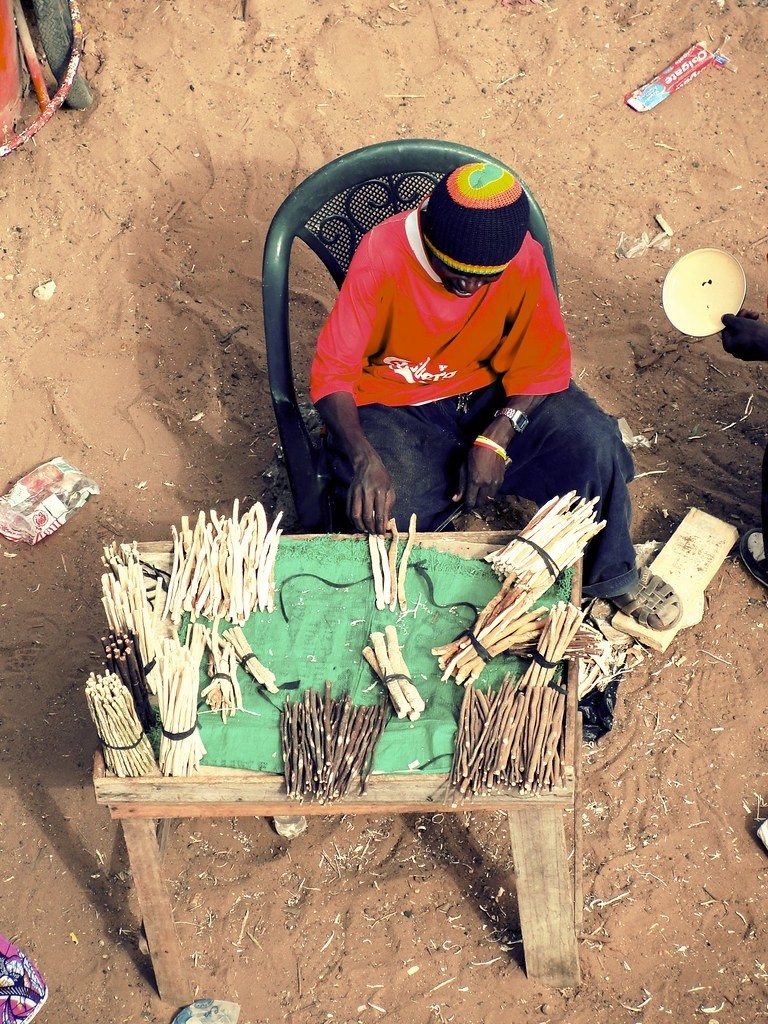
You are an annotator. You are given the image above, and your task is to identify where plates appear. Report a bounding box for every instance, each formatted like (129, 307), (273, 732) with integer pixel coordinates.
(662, 249), (746, 337)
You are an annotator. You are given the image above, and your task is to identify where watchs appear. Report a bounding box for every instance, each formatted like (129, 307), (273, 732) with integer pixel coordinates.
(494, 408), (530, 434)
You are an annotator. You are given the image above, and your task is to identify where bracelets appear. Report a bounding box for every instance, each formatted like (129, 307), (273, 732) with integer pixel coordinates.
(474, 435), (512, 465)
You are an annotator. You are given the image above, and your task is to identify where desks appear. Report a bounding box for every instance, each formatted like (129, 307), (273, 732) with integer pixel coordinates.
(92, 530), (585, 986)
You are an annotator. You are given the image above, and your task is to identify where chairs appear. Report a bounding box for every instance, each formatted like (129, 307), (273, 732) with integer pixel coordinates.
(260, 137), (560, 529)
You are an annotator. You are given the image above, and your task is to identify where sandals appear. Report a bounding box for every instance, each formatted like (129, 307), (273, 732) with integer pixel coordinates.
(611, 566), (683, 631)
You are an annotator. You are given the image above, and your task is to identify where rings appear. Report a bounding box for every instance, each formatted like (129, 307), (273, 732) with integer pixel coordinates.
(486, 495), (494, 500)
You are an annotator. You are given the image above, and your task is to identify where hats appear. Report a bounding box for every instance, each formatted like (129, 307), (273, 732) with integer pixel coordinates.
(423, 161), (530, 278)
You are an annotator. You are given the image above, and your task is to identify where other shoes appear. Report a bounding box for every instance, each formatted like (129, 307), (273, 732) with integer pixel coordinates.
(739, 527), (768, 588)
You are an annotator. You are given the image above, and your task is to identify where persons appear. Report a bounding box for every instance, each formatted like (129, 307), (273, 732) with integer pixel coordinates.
(721, 308), (768, 587)
(307, 161), (685, 635)
